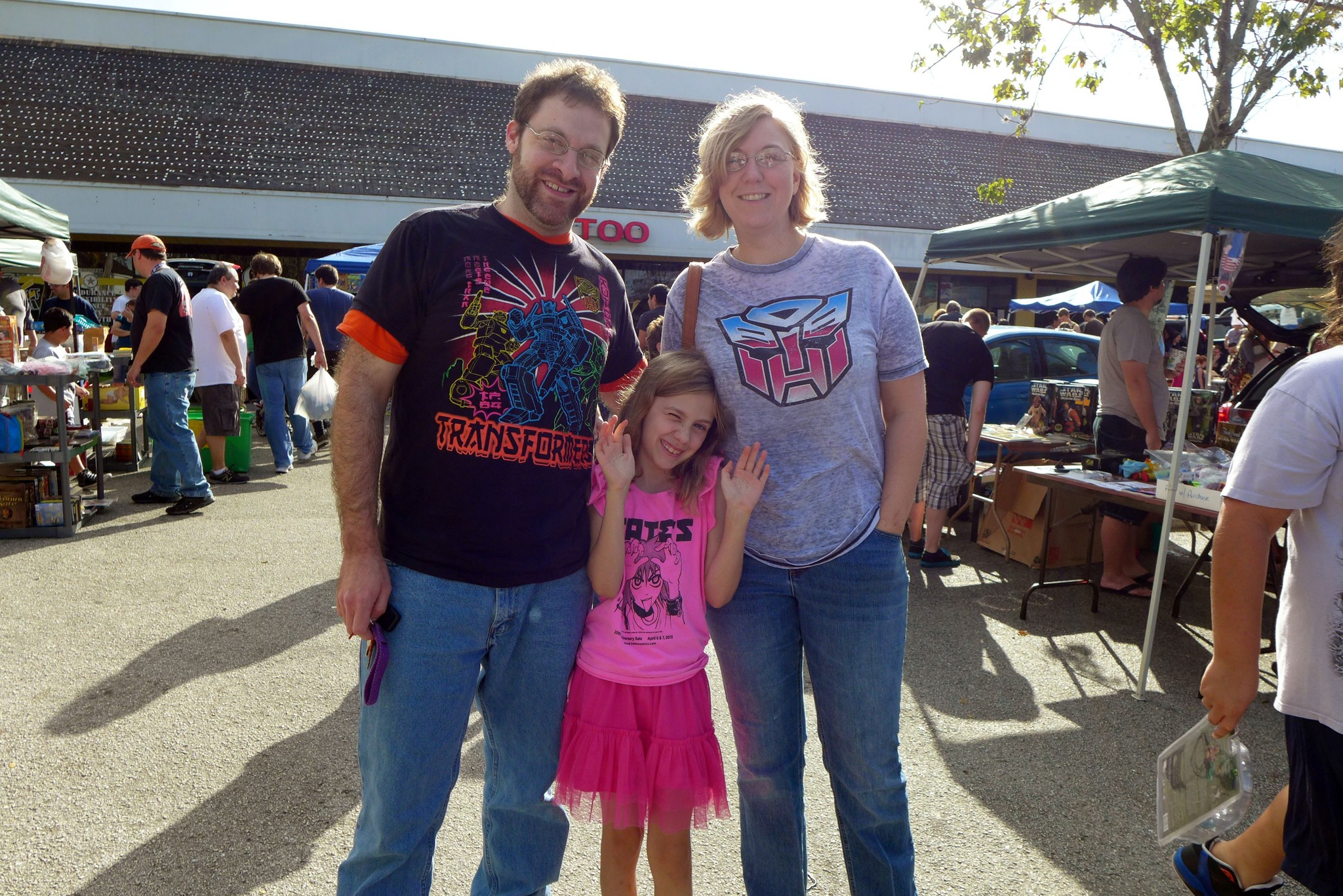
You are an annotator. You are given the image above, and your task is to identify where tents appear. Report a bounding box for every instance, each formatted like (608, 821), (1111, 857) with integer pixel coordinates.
(303, 242), (386, 292)
(910, 149), (1343, 701)
(1006, 280), (1126, 322)
(0, 238), (82, 298)
(0, 178), (83, 427)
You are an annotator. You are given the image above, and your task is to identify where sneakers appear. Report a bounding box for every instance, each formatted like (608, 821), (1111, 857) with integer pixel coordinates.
(132, 490), (181, 503)
(209, 467), (250, 484)
(275, 464), (293, 473)
(165, 495), (216, 514)
(1172, 836), (1283, 896)
(297, 440), (318, 460)
(316, 434), (329, 450)
(77, 468), (112, 490)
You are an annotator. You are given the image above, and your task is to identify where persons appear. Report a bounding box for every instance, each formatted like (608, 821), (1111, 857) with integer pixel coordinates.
(661, 87), (931, 895)
(1094, 255), (1170, 599)
(645, 314), (664, 361)
(1188, 395), (1209, 434)
(0, 253), (356, 489)
(330, 61), (651, 896)
(636, 284), (671, 353)
(554, 346), (770, 896)
(930, 299), (1323, 404)
(121, 235), (215, 516)
(905, 308), (995, 569)
(1172, 221), (1343, 896)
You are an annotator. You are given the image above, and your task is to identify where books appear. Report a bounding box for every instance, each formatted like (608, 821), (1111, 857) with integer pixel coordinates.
(0, 465), (114, 529)
(1028, 378), (1100, 436)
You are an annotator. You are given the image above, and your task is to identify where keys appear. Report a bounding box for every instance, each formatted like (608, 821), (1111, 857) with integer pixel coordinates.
(349, 603), (403, 669)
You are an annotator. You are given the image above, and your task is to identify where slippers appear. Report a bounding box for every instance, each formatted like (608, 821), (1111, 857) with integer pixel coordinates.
(1133, 572), (1167, 587)
(1099, 581), (1152, 599)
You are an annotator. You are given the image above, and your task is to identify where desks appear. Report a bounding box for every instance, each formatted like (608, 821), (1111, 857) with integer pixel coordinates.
(1012, 464), (1287, 623)
(945, 423), (1096, 563)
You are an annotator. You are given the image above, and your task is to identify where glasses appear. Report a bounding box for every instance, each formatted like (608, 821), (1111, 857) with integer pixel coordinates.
(725, 147), (798, 171)
(524, 122), (610, 170)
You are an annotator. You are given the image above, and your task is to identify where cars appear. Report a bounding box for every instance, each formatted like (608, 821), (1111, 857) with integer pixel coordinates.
(963, 323), (1100, 461)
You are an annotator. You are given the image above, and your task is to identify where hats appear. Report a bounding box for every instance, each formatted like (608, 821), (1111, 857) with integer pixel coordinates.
(126, 235), (167, 259)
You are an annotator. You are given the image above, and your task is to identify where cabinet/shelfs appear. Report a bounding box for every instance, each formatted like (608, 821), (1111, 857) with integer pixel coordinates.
(78, 370), (150, 472)
(0, 369), (107, 538)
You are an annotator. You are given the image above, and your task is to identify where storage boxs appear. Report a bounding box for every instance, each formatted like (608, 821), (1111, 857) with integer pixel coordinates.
(0, 314), (21, 363)
(187, 406), (244, 413)
(1082, 452), (1137, 475)
(152, 412), (255, 473)
(83, 325), (110, 353)
(1160, 386), (1221, 444)
(90, 426), (128, 446)
(1156, 477), (1223, 512)
(976, 458), (1163, 571)
